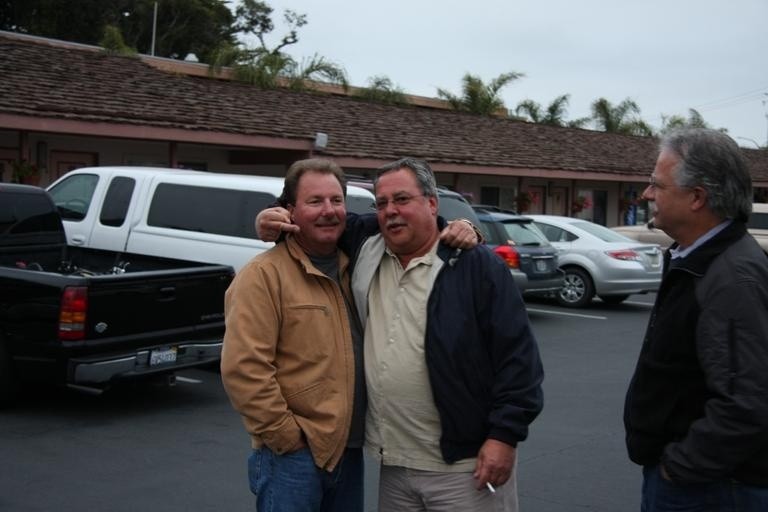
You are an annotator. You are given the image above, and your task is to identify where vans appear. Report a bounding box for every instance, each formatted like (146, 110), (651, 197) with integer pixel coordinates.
(44, 165), (379, 276)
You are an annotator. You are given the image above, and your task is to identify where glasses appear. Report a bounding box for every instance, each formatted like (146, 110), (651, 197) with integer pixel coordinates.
(368, 192), (427, 211)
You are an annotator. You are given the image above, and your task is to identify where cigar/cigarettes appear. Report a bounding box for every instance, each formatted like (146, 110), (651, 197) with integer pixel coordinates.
(486, 479), (499, 496)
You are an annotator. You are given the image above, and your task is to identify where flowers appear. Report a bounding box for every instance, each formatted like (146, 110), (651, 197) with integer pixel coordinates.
(577, 192), (592, 208)
(511, 190), (540, 206)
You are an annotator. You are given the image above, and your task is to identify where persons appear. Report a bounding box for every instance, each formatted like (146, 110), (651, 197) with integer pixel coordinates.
(622, 126), (768, 512)
(214, 158), (485, 512)
(252, 160), (545, 511)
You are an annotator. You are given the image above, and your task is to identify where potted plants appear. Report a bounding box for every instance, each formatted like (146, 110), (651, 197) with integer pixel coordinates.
(8, 158), (49, 187)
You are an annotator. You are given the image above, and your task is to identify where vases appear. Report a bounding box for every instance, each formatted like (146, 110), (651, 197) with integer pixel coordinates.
(518, 202), (528, 211)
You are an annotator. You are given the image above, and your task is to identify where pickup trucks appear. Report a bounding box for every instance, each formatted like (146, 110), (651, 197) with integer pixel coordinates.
(0, 183), (236, 395)
